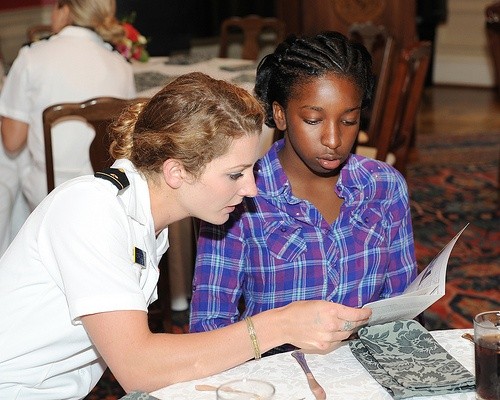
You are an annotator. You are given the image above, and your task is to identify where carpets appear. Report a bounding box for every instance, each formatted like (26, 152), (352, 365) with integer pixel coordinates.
(407, 129), (500, 329)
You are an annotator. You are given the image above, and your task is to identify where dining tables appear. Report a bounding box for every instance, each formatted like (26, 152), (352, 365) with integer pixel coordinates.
(150, 322), (500, 400)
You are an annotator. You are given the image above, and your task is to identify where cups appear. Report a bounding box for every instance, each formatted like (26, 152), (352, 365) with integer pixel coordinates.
(216, 379), (275, 400)
(473, 310), (500, 400)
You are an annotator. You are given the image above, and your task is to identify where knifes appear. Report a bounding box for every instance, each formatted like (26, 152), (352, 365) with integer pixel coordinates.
(292, 350), (327, 400)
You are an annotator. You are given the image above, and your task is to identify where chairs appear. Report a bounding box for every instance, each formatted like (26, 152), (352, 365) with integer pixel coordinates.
(362, 37), (434, 169)
(220, 14), (290, 66)
(345, 22), (399, 130)
(40, 93), (146, 197)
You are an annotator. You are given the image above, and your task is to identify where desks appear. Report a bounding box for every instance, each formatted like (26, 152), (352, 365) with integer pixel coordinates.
(132, 53), (260, 96)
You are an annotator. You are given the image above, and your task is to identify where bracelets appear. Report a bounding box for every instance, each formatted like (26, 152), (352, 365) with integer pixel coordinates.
(246, 316), (262, 360)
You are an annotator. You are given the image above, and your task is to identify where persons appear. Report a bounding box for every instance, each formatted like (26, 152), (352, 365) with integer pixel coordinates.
(190, 34), (417, 350)
(0, 0), (136, 214)
(0, 71), (373, 399)
(382, 0), (447, 89)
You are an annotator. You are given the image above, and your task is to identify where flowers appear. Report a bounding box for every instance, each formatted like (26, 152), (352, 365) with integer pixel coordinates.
(111, 18), (150, 62)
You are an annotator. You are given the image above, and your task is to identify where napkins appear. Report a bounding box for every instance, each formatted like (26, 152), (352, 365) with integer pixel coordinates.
(134, 71), (178, 91)
(165, 54), (211, 64)
(349, 317), (476, 400)
(218, 63), (257, 70)
(233, 72), (256, 82)
(119, 388), (161, 400)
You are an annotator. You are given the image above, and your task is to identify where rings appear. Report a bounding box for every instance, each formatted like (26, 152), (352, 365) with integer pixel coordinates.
(344, 320), (351, 331)
